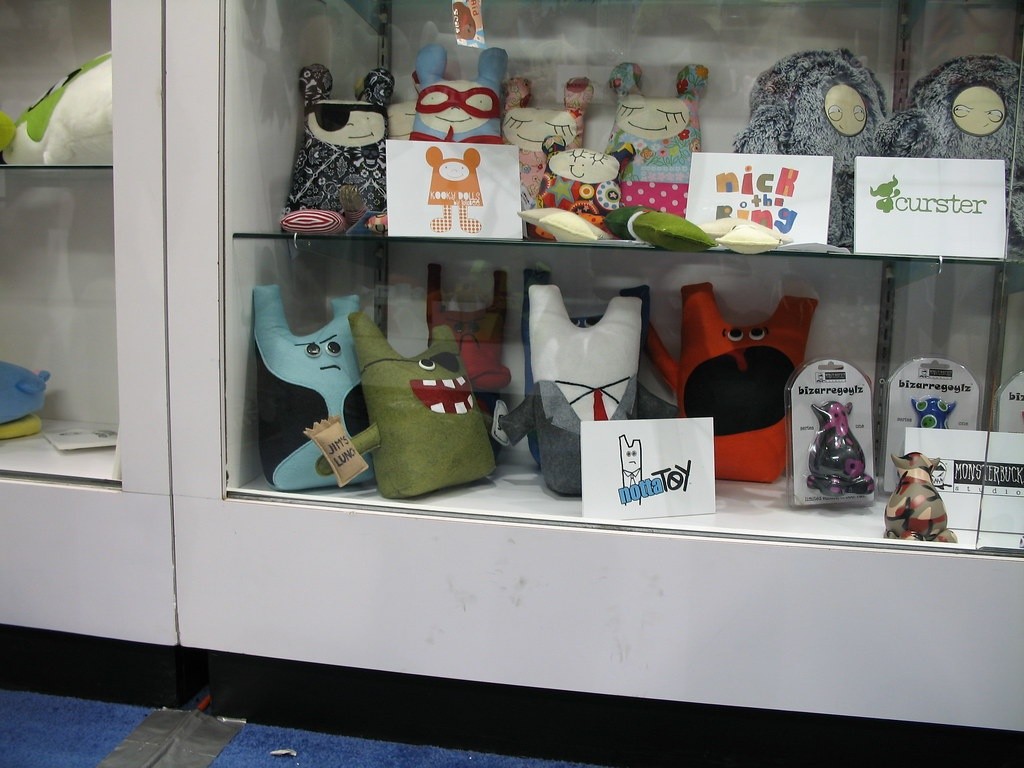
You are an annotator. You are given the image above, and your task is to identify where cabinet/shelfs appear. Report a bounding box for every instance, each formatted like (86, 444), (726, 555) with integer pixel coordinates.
(2, 0), (1024, 729)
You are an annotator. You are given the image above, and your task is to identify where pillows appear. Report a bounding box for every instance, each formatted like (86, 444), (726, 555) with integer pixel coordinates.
(3, 47), (114, 166)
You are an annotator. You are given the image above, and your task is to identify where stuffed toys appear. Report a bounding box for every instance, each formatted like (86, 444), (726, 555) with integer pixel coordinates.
(278, 45), (1024, 253)
(0, 54), (113, 166)
(255, 262), (818, 498)
